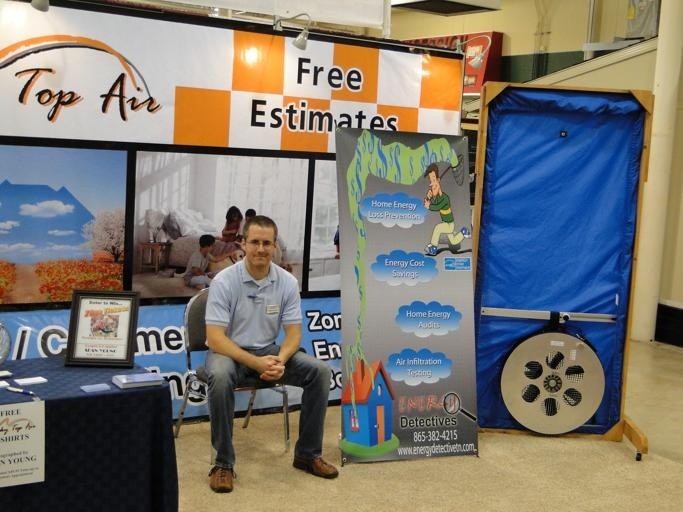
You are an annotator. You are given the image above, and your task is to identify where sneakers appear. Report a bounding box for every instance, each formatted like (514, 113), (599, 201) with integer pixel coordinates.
(209, 464), (236, 493)
(424, 243), (438, 256)
(460, 226), (472, 239)
(293, 454), (339, 479)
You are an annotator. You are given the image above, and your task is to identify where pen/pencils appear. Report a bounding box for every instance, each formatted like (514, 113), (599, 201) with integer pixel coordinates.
(6, 386), (36, 396)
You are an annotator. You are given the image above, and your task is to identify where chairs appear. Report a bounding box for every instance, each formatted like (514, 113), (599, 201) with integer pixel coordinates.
(171, 287), (289, 461)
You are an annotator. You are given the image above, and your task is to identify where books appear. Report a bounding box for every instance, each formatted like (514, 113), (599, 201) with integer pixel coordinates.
(110, 373), (165, 388)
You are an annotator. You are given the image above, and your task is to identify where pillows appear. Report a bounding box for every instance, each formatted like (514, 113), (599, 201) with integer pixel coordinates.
(163, 208), (217, 240)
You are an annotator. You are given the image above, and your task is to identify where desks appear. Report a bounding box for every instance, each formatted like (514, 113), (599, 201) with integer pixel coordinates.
(137, 242), (168, 276)
(0, 357), (172, 511)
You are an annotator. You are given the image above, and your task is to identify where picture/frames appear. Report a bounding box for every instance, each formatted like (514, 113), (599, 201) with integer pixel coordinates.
(0, 135), (341, 311)
(62, 289), (140, 370)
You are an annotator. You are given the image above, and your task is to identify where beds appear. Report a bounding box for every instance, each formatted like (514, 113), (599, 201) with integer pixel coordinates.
(142, 229), (281, 274)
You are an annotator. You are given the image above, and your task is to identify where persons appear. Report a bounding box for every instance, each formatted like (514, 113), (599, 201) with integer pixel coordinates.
(333, 224), (340, 259)
(181, 204), (293, 290)
(204, 215), (339, 490)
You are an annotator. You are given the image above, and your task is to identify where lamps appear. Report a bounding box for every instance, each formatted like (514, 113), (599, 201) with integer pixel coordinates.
(454, 34), (493, 70)
(269, 13), (312, 51)
